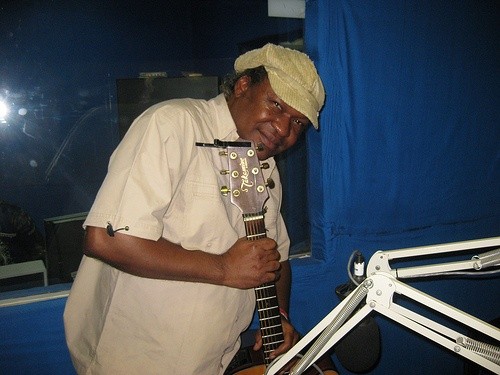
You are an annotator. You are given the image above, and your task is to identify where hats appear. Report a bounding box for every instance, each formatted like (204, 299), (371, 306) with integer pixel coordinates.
(234, 42), (326, 130)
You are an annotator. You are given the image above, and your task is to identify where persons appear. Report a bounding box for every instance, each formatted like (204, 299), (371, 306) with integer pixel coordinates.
(62, 44), (325, 375)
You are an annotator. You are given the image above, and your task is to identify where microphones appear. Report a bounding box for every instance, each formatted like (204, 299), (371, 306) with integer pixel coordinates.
(335, 252), (382, 373)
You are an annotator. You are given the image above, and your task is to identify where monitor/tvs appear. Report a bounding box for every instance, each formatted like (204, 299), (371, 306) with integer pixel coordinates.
(44, 211), (90, 286)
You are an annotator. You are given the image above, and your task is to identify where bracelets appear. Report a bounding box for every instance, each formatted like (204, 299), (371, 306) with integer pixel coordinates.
(279, 307), (289, 321)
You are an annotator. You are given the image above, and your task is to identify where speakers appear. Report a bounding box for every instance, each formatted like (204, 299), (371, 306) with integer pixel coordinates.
(108, 75), (218, 149)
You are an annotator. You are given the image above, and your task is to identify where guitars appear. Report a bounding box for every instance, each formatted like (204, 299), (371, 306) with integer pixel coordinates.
(218, 138), (336, 375)
